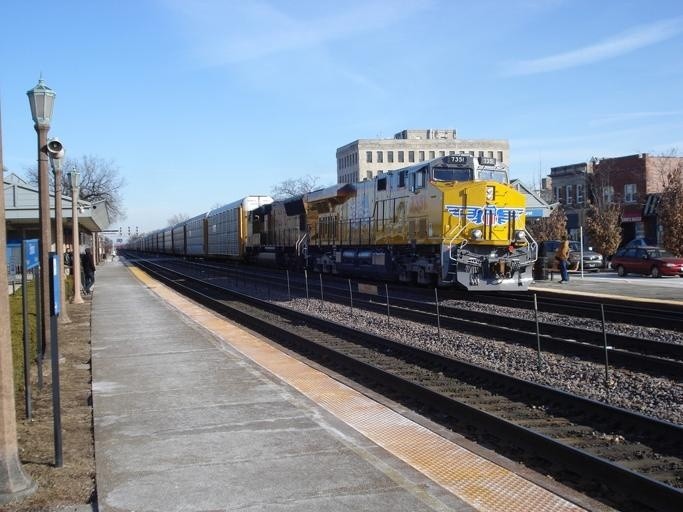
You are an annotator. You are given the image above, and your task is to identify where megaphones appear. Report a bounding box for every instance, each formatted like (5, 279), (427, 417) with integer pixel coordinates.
(47, 137), (63, 152)
(50, 150), (63, 158)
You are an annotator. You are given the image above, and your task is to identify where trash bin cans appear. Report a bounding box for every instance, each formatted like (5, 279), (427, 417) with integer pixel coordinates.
(532, 256), (549, 280)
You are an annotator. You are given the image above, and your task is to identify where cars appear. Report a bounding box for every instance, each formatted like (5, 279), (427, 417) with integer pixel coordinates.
(611, 246), (683, 278)
(538, 240), (603, 269)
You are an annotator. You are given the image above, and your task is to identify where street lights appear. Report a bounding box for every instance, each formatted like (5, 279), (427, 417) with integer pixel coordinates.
(26, 71), (84, 361)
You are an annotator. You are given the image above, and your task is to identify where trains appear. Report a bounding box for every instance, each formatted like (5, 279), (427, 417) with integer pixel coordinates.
(130, 154), (538, 291)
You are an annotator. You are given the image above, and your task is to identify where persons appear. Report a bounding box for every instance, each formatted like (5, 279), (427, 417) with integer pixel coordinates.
(63, 246), (72, 275)
(81, 247), (95, 295)
(554, 236), (569, 282)
